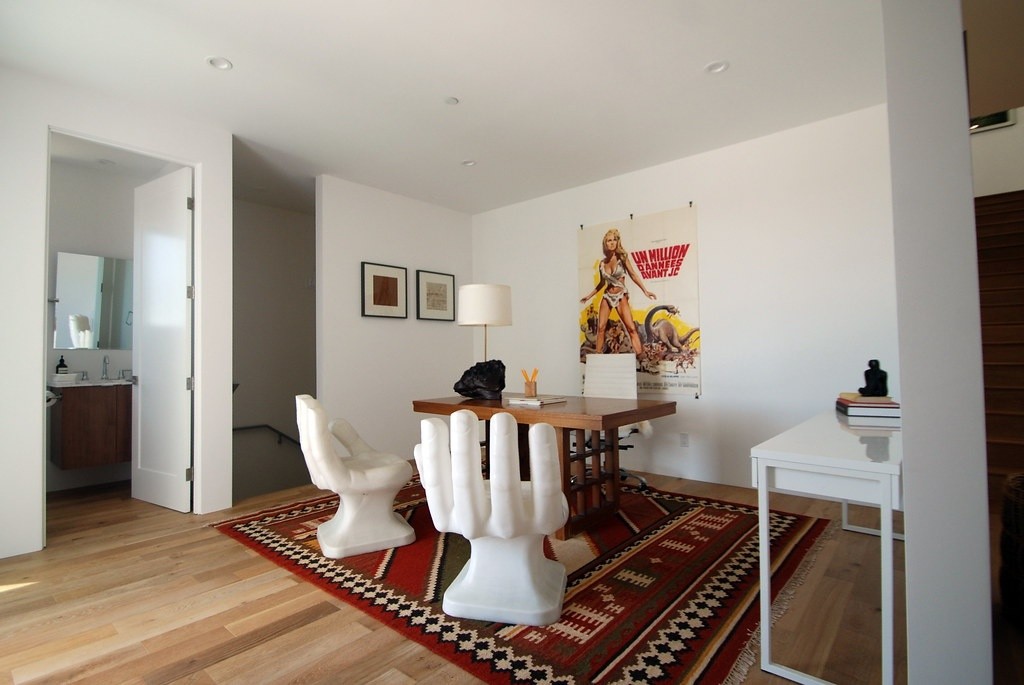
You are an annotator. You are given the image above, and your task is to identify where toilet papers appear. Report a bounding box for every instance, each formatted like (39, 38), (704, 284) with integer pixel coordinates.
(46, 390), (57, 408)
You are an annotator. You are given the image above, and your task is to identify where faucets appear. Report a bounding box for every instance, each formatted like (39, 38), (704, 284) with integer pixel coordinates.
(102, 354), (110, 379)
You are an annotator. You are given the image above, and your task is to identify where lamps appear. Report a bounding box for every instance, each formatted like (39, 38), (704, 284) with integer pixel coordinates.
(458, 284), (513, 362)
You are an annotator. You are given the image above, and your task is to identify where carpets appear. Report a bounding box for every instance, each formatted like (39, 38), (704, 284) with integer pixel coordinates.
(205, 457), (842, 685)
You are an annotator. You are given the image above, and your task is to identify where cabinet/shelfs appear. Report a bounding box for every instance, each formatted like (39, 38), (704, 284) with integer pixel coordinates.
(49, 385), (132, 472)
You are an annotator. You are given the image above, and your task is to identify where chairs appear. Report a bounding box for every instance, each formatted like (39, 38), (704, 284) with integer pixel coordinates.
(414, 409), (570, 626)
(571, 353), (648, 491)
(69, 315), (90, 347)
(295, 394), (417, 559)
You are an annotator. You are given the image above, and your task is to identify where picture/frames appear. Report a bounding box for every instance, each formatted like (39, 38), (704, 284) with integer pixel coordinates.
(361, 261), (407, 319)
(416, 270), (455, 321)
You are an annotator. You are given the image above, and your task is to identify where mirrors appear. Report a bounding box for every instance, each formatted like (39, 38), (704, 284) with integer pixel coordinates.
(53, 251), (134, 350)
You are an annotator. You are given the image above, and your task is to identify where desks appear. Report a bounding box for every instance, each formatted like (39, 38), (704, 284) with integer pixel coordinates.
(751, 409), (903, 685)
(413, 392), (677, 542)
